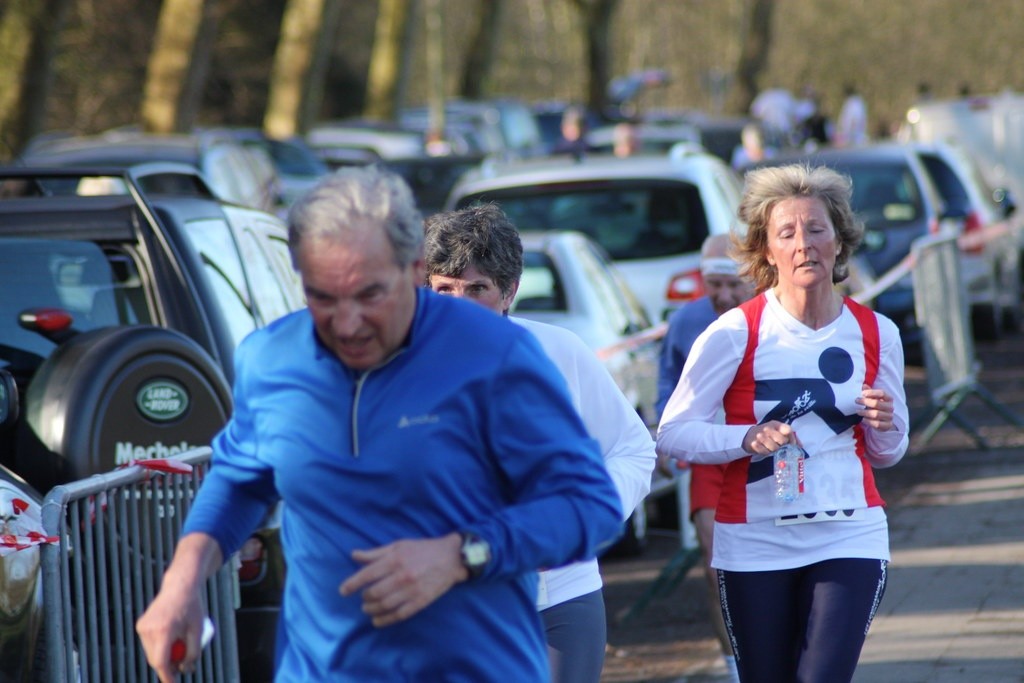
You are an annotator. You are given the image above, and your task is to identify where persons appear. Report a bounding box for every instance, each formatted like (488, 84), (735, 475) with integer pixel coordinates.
(541, 70), (1023, 174)
(134, 161), (624, 683)
(655, 161), (910, 683)
(648, 235), (760, 683)
(419, 200), (657, 682)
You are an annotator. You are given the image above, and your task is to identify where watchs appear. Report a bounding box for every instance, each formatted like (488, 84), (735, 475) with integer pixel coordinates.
(453, 531), (492, 586)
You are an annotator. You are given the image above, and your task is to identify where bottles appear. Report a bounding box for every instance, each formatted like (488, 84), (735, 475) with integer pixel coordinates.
(773, 439), (805, 501)
(170, 616), (215, 663)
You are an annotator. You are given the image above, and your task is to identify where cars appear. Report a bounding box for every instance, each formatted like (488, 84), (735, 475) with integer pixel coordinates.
(0, 97), (1023, 682)
